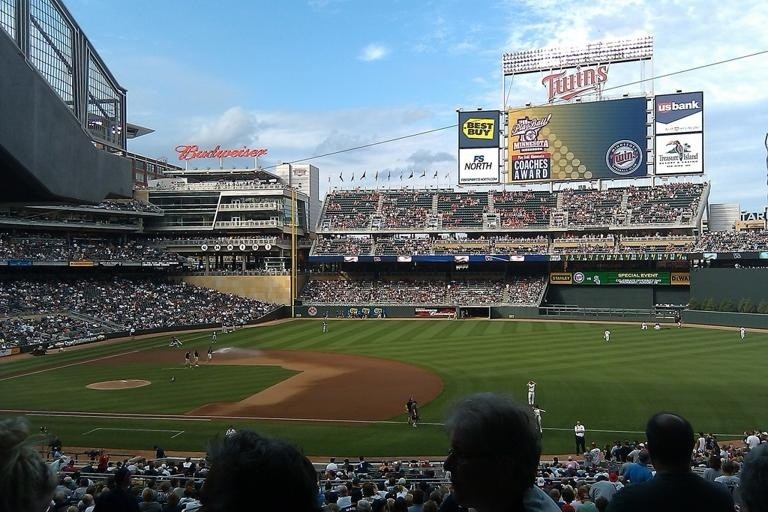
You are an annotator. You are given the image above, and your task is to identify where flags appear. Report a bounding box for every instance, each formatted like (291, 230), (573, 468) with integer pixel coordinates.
(339, 170), (451, 180)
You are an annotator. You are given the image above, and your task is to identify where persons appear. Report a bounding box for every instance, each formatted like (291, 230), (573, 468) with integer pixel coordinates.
(191, 349), (200, 368)
(652, 322), (660, 329)
(603, 328), (611, 344)
(526, 380), (537, 413)
(322, 320), (329, 334)
(738, 325), (745, 342)
(406, 396), (414, 425)
(533, 405), (546, 440)
(206, 346), (214, 363)
(640, 321), (648, 330)
(675, 321), (681, 328)
(184, 351), (190, 368)
(441, 387), (559, 512)
(411, 401), (418, 428)
